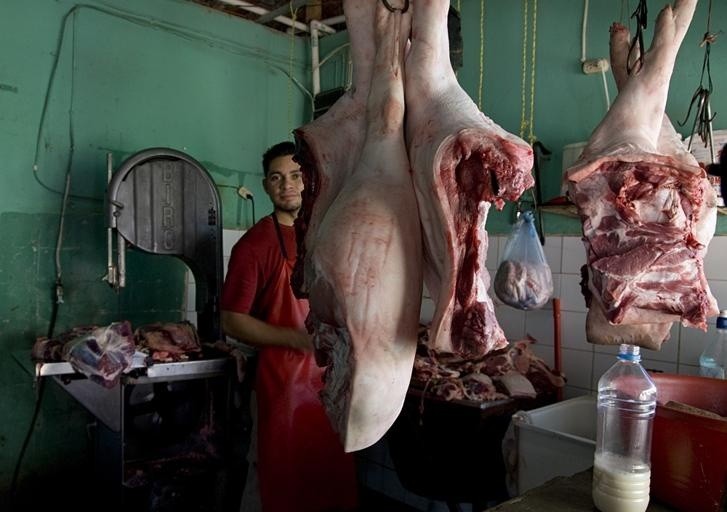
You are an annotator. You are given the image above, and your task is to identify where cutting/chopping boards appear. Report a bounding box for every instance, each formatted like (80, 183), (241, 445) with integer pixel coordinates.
(410, 386), (559, 415)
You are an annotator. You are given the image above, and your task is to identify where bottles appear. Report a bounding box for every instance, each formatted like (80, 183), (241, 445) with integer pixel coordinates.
(699, 310), (727, 380)
(593, 345), (654, 508)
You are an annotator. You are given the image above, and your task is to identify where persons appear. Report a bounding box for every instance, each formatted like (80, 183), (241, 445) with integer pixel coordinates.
(217, 141), (358, 511)
